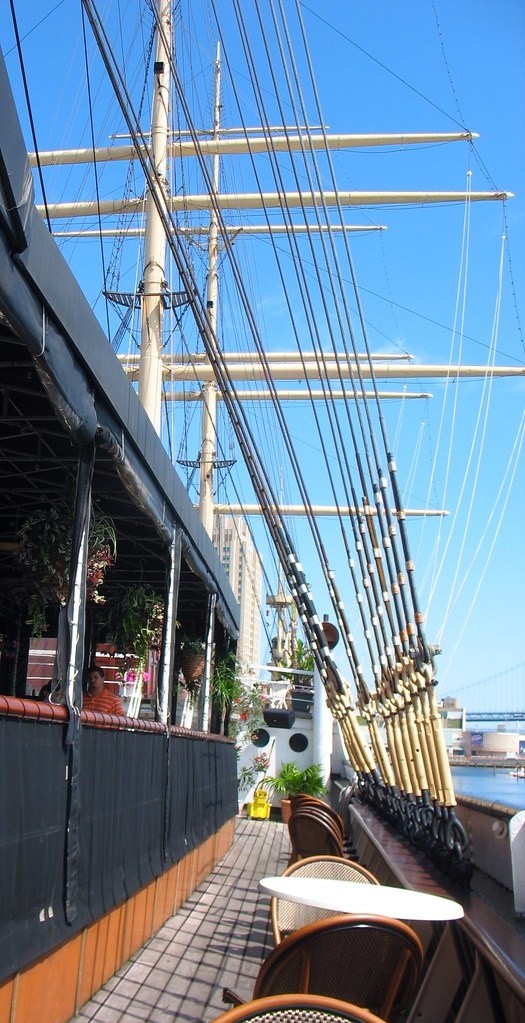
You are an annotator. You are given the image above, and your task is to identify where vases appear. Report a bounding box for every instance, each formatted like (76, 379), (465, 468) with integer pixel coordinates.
(236, 800), (244, 815)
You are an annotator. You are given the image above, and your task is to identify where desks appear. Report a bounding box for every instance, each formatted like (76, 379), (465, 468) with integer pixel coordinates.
(260, 877), (465, 920)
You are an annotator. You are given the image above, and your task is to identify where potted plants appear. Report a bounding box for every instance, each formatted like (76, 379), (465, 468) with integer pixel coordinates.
(265, 760), (328, 822)
(277, 639), (314, 711)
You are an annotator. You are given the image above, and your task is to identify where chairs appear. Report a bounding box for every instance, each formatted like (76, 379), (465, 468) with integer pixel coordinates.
(208, 793), (425, 1023)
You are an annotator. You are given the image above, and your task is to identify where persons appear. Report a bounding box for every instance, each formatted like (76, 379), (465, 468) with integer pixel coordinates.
(43, 679), (61, 703)
(82, 665), (124, 716)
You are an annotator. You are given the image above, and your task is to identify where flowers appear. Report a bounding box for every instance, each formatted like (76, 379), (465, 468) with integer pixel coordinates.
(18, 504), (117, 643)
(175, 637), (241, 709)
(97, 583), (182, 686)
